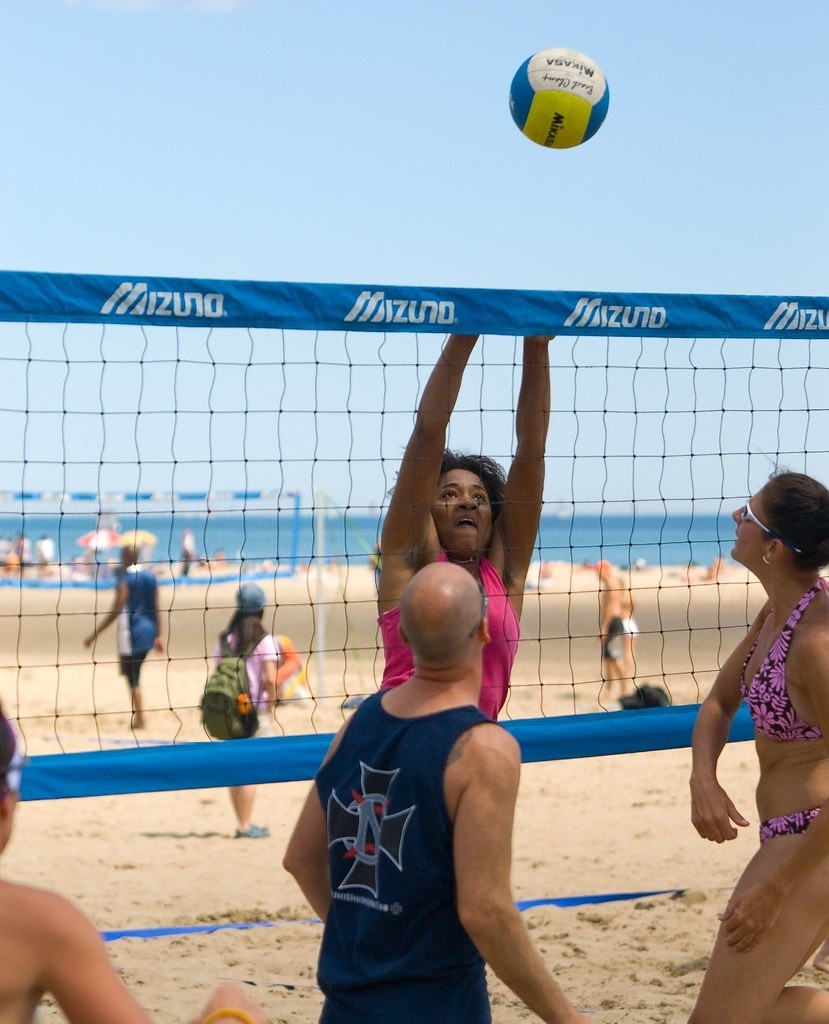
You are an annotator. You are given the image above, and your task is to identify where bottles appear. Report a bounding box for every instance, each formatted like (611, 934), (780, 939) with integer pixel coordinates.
(237, 694), (252, 715)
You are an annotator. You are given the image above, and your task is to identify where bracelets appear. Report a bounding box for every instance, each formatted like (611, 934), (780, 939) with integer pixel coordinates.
(203, 1007), (254, 1024)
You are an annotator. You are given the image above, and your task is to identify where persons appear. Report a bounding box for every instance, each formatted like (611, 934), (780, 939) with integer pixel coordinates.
(689, 473), (829, 1024)
(1, 708), (269, 1024)
(283, 562), (598, 1024)
(212, 582), (309, 839)
(540, 551), (727, 594)
(85, 545), (163, 728)
(377, 334), (553, 725)
(595, 560), (638, 701)
(1, 527), (269, 581)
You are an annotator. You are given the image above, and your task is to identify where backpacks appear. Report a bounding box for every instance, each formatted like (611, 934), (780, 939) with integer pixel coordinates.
(201, 632), (270, 740)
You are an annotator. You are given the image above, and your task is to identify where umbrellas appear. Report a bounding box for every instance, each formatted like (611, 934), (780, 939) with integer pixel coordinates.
(76, 529), (121, 548)
(116, 530), (156, 546)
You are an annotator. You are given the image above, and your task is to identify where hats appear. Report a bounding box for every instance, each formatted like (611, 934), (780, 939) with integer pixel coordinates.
(237, 582), (264, 613)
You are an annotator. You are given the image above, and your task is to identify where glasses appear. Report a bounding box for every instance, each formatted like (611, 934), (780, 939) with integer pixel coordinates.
(741, 501), (802, 556)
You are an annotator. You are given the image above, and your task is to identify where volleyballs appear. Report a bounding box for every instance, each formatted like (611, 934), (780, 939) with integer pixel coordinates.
(508, 46), (611, 150)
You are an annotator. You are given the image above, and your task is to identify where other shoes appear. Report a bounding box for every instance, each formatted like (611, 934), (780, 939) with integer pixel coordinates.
(237, 825), (271, 839)
